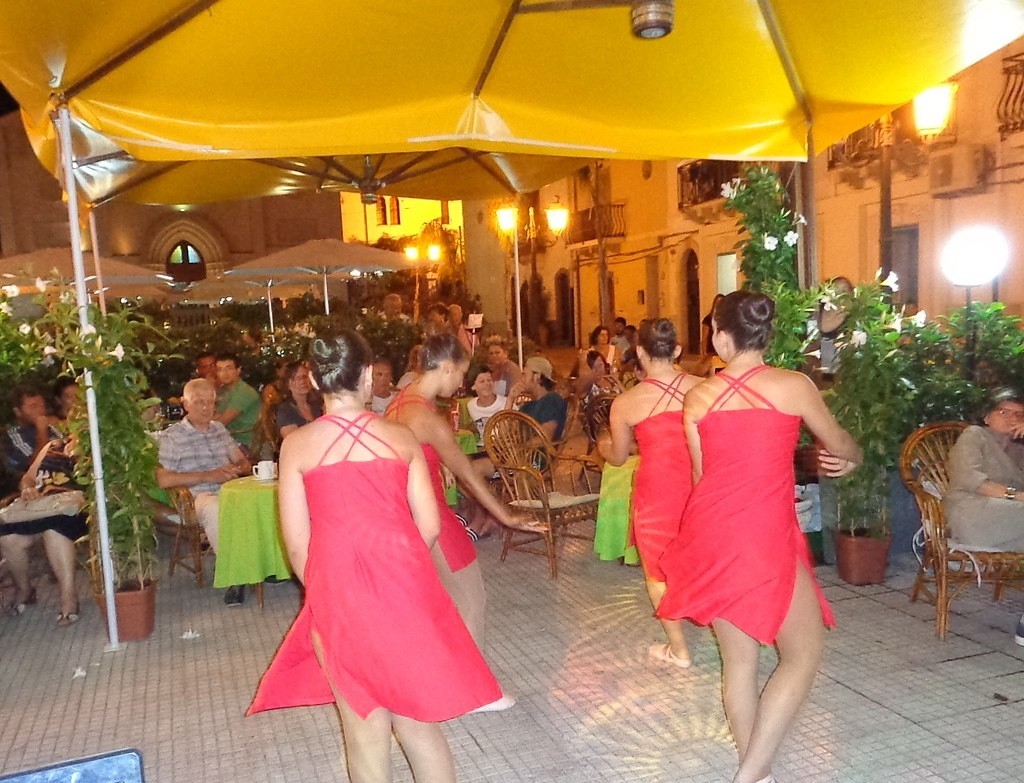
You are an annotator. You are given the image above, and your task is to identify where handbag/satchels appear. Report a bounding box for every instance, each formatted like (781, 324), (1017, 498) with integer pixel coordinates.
(1, 488), (84, 525)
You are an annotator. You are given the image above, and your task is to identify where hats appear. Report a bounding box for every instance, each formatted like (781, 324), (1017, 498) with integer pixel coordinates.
(526, 357), (556, 383)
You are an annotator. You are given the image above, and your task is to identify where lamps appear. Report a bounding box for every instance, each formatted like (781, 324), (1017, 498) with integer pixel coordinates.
(913, 80), (960, 149)
(544, 196), (569, 243)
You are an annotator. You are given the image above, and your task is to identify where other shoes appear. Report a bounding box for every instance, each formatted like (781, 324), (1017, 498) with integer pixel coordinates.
(1014, 617), (1024, 646)
(223, 584), (243, 606)
(265, 575), (288, 585)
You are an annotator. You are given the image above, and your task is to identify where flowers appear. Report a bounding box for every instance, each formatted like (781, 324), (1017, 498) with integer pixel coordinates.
(1, 268), (161, 589)
(808, 273), (936, 537)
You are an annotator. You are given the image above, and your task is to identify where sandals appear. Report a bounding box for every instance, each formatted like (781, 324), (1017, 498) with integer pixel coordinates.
(9, 587), (37, 616)
(56, 597), (79, 626)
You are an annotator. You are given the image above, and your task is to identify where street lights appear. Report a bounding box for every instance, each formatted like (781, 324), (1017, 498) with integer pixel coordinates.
(495, 205), (569, 350)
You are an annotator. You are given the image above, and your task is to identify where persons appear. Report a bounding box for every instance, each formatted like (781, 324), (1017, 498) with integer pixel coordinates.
(0, 377), (88, 625)
(598, 318), (709, 668)
(571, 317), (647, 408)
(366, 295), (568, 542)
(382, 321), (553, 712)
(946, 390), (1024, 647)
(701, 295), (724, 357)
(820, 278), (853, 338)
(138, 349), (325, 605)
(245, 329), (502, 783)
(654, 289), (865, 783)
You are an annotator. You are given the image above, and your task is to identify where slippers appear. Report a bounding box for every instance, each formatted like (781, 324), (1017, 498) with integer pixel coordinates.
(465, 527), (481, 541)
(455, 513), (468, 527)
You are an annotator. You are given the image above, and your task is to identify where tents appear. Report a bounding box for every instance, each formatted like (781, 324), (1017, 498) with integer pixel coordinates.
(0, 0), (1022, 652)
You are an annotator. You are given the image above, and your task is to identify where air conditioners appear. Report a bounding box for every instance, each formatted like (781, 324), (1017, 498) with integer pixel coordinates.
(927, 143), (986, 194)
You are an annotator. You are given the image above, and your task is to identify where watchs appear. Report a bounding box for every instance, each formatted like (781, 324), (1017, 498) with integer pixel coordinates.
(1006, 486), (1017, 499)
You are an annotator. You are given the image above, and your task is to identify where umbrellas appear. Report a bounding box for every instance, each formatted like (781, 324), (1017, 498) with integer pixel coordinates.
(223, 239), (421, 315)
(0, 248), (175, 304)
(179, 270), (321, 345)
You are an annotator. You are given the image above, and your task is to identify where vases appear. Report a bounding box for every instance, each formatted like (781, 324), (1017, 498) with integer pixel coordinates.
(96, 578), (156, 641)
(833, 528), (896, 587)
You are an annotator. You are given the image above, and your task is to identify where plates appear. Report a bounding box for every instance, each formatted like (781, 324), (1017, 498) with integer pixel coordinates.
(251, 473), (277, 482)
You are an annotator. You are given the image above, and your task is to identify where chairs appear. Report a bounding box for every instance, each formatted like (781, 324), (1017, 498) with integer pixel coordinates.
(455, 371), (642, 580)
(163, 397), (288, 592)
(900, 422), (1023, 643)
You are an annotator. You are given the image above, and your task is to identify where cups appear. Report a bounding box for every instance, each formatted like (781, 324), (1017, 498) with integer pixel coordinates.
(253, 460), (275, 478)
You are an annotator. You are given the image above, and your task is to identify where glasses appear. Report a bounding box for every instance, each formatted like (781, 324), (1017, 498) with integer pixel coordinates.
(993, 407), (1024, 420)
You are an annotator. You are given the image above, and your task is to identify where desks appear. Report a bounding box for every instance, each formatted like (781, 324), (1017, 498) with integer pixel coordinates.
(593, 456), (639, 569)
(218, 475), (292, 610)
